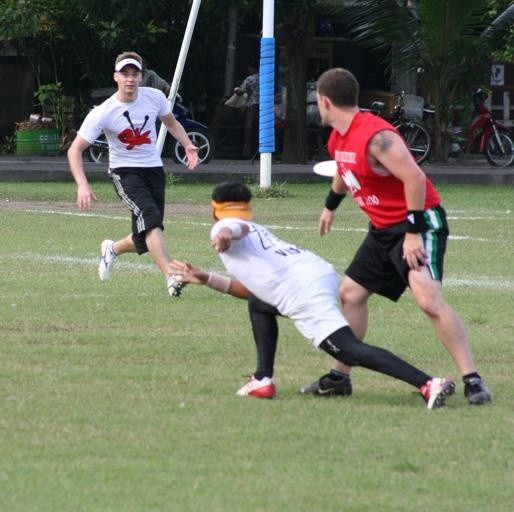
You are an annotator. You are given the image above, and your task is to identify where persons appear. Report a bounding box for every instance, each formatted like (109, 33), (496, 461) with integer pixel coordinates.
(137, 58), (169, 97)
(66, 52), (199, 297)
(272, 86), (287, 128)
(298, 67), (491, 406)
(233, 57), (260, 158)
(165, 180), (454, 410)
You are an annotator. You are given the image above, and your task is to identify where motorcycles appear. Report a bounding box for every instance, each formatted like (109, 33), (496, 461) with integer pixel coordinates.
(87, 92), (216, 168)
(439, 87), (513, 166)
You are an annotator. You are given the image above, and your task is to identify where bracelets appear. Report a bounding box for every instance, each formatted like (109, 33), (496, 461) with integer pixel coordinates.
(324, 187), (346, 211)
(203, 272), (232, 294)
(405, 210), (423, 235)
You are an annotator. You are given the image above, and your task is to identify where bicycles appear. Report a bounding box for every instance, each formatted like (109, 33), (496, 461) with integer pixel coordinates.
(372, 90), (433, 165)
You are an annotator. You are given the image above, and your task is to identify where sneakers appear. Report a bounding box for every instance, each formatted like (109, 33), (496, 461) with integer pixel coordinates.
(299, 375), (353, 397)
(99, 239), (118, 282)
(418, 377), (456, 411)
(462, 374), (491, 405)
(167, 272), (185, 298)
(236, 374), (277, 399)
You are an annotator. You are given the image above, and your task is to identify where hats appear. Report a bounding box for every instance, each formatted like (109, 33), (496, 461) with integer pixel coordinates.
(210, 200), (254, 221)
(115, 57), (143, 71)
